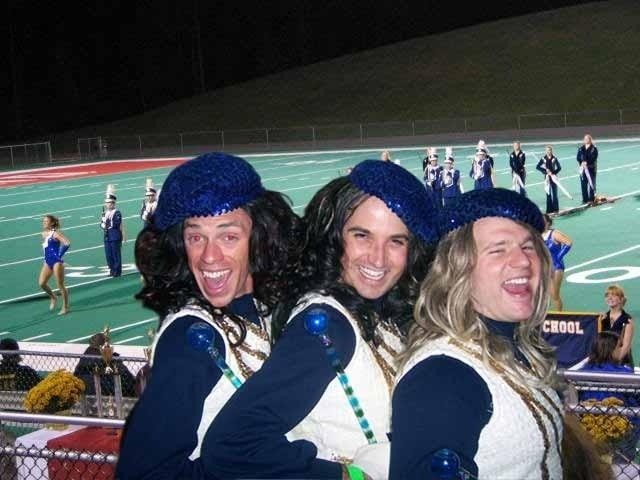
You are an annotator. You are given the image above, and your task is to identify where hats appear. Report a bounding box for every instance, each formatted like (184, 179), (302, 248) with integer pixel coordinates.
(347, 158), (435, 242)
(429, 153), (438, 161)
(445, 155), (454, 163)
(150, 150), (267, 230)
(104, 193), (116, 203)
(145, 188), (156, 197)
(436, 187), (544, 233)
(475, 148), (486, 156)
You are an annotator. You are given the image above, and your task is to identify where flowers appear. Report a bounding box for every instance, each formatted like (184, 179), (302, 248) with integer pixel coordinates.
(22, 368), (90, 415)
(572, 397), (631, 458)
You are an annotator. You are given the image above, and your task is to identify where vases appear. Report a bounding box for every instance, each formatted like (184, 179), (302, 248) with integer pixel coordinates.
(45, 409), (73, 433)
(595, 448), (617, 468)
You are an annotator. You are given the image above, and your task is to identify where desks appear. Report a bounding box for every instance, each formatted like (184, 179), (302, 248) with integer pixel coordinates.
(13, 420), (125, 480)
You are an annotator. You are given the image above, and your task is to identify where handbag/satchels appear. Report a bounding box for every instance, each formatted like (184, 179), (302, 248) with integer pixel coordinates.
(564, 415), (612, 480)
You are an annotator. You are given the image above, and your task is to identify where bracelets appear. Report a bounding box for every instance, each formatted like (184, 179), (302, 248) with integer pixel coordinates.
(345, 464), (365, 480)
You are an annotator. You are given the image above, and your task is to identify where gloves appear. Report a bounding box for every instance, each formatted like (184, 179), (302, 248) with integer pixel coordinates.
(101, 217), (107, 229)
(142, 211), (148, 220)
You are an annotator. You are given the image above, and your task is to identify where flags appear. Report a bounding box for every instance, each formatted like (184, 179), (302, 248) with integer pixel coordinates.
(537, 311), (603, 371)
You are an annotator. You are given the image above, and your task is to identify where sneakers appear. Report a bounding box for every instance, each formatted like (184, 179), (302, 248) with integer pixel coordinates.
(57, 308), (70, 316)
(49, 297), (58, 311)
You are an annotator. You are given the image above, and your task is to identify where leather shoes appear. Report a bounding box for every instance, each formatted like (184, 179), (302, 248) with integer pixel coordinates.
(108, 272), (122, 277)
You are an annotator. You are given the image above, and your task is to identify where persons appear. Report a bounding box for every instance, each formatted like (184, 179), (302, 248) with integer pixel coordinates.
(421, 154), (441, 207)
(469, 147), (493, 190)
(199, 157), (437, 480)
(575, 329), (640, 464)
(509, 141), (526, 198)
(139, 188), (157, 228)
(74, 334), (133, 395)
(111, 149), (300, 480)
(385, 186), (571, 480)
(541, 214), (572, 311)
(535, 145), (562, 214)
(438, 157), (461, 209)
(38, 214), (72, 316)
(100, 190), (124, 278)
(0, 339), (39, 391)
(598, 311), (608, 322)
(602, 285), (634, 367)
(576, 134), (598, 205)
